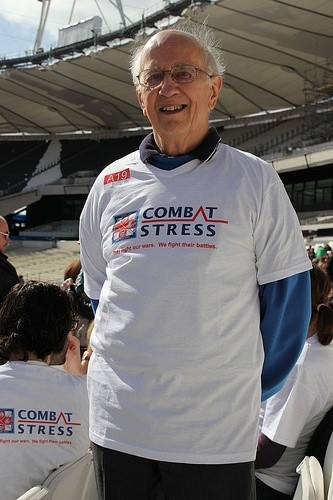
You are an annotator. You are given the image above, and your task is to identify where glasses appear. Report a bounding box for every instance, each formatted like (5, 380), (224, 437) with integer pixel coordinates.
(0, 231), (10, 239)
(71, 319), (79, 330)
(136, 65), (211, 88)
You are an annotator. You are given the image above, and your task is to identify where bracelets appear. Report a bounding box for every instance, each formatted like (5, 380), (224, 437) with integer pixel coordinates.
(82, 350), (92, 362)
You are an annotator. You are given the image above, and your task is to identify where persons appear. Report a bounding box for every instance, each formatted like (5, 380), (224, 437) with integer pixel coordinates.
(306, 240), (333, 307)
(79, 27), (316, 500)
(0, 279), (90, 500)
(0, 215), (96, 375)
(255, 262), (333, 500)
